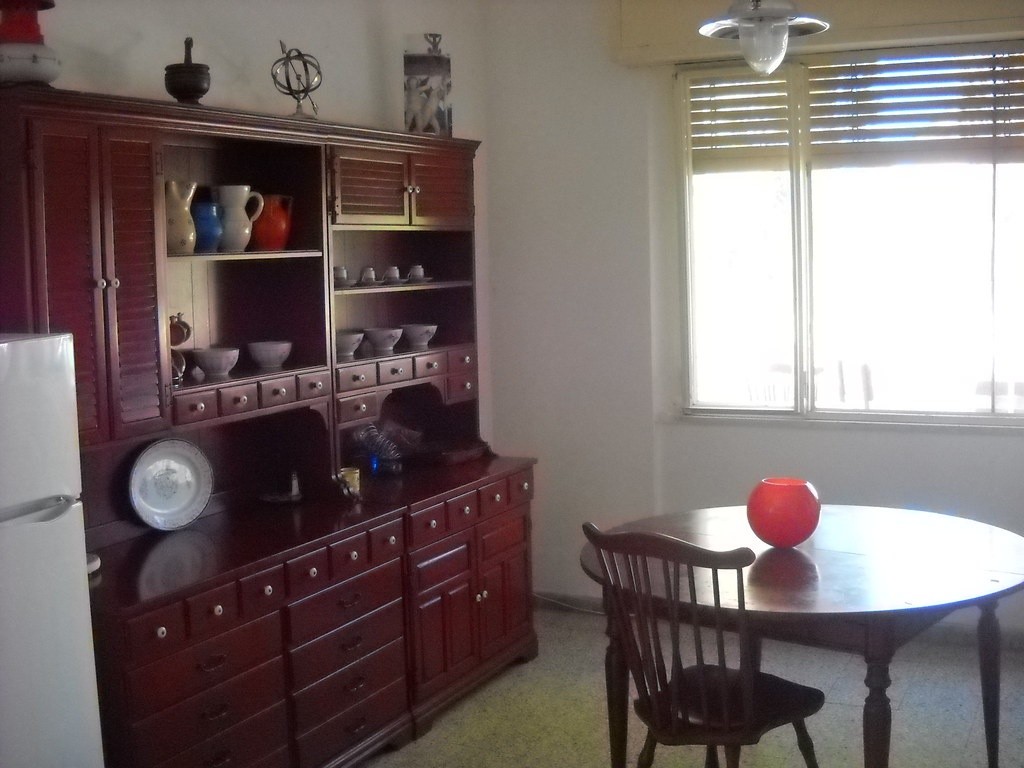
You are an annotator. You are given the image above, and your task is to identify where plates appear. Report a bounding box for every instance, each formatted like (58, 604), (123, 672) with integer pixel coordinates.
(128, 438), (214, 531)
(375, 280), (384, 285)
(408, 276), (432, 283)
(384, 279), (408, 285)
(347, 280), (357, 286)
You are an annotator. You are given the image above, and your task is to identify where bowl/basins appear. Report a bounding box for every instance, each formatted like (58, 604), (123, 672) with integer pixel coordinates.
(247, 341), (293, 368)
(362, 328), (404, 351)
(191, 348), (239, 375)
(336, 331), (363, 356)
(400, 324), (438, 345)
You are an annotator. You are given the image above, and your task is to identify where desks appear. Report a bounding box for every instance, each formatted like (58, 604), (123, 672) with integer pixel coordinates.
(576, 502), (1024, 767)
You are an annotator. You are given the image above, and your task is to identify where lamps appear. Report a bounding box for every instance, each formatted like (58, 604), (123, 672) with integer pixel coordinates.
(699, 0), (831, 76)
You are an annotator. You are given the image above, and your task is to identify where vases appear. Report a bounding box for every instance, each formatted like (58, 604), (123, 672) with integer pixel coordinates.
(210, 184), (265, 252)
(252, 194), (294, 248)
(749, 478), (819, 549)
(164, 180), (198, 254)
(188, 201), (224, 252)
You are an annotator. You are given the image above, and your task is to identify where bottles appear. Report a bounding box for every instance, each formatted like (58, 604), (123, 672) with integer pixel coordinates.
(249, 195), (294, 251)
(165, 180), (197, 255)
(191, 186), (225, 253)
(219, 186), (264, 253)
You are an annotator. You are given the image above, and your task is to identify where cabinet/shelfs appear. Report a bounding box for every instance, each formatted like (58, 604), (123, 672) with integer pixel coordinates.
(0, 82), (538, 768)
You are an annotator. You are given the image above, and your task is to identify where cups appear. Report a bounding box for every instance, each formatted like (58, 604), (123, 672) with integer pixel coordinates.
(360, 268), (375, 286)
(334, 266), (349, 288)
(408, 264), (424, 278)
(381, 267), (400, 280)
(342, 467), (360, 496)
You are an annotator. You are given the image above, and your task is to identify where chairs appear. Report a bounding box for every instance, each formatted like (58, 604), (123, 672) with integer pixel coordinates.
(581, 522), (824, 768)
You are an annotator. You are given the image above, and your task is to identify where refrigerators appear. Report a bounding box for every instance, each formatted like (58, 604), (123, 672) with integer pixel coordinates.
(0, 332), (105, 768)
